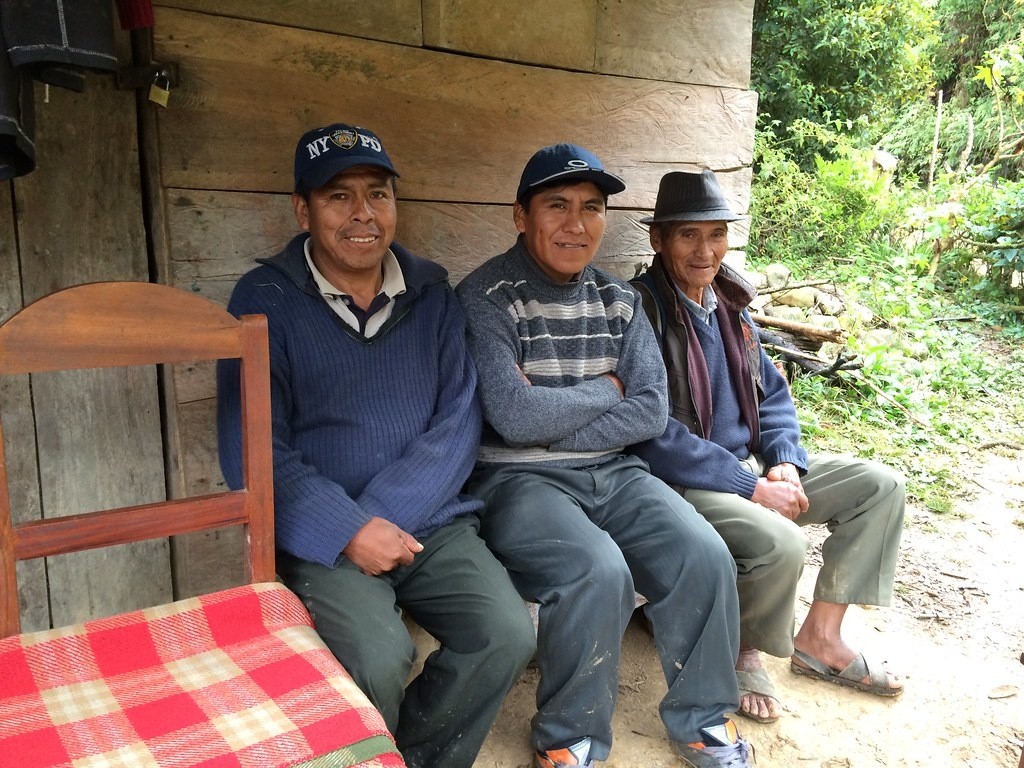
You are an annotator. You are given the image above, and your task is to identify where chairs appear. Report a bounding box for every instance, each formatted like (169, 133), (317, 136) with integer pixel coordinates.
(0, 281), (406, 768)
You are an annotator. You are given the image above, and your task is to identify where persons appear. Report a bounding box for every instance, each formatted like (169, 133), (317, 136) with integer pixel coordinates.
(217, 122), (538, 768)
(627, 171), (906, 725)
(454, 143), (757, 768)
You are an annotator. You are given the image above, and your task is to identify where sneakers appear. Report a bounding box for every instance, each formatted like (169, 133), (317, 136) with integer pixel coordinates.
(672, 723), (757, 768)
(533, 736), (593, 768)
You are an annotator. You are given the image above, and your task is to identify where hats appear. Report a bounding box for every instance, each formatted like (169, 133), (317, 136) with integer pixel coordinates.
(517, 144), (627, 202)
(294, 123), (401, 194)
(640, 170), (746, 224)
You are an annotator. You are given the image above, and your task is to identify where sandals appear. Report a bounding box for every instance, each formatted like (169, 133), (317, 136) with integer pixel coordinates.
(735, 667), (781, 726)
(791, 647), (903, 697)
